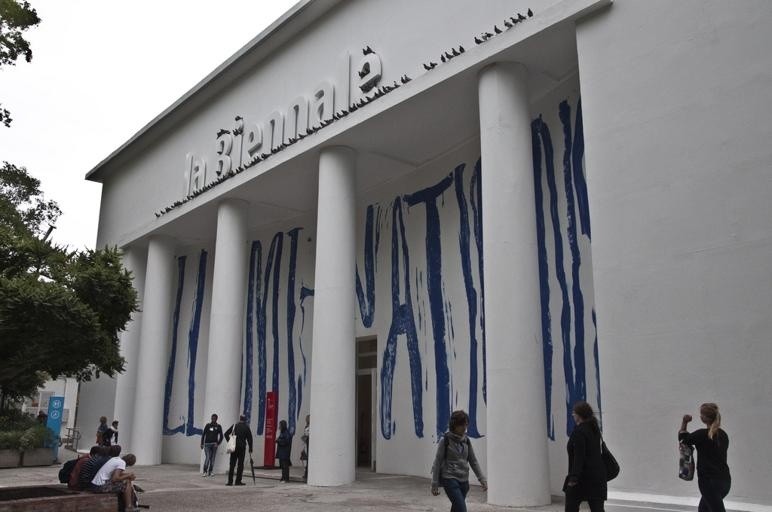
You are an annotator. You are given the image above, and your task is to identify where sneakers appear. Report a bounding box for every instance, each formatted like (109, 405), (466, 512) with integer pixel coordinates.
(201, 472), (207, 477)
(209, 473), (214, 477)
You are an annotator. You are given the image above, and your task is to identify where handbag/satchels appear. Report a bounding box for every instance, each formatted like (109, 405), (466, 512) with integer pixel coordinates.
(602, 441), (620, 482)
(678, 441), (695, 481)
(226, 434), (237, 454)
(562, 473), (590, 499)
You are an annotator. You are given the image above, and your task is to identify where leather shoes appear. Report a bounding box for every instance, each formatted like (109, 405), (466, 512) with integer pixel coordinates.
(235, 481), (246, 485)
(225, 482), (232, 486)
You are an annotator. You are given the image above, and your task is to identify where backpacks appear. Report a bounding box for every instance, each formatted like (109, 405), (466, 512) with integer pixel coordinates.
(58, 459), (78, 483)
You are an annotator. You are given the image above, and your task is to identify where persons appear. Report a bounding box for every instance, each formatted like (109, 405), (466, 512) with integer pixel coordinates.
(58, 444), (140, 511)
(677, 403), (732, 511)
(274, 419), (292, 484)
(95, 415), (119, 447)
(429, 411), (488, 512)
(200, 413), (223, 479)
(299, 414), (309, 481)
(561, 400), (620, 511)
(225, 414), (257, 486)
